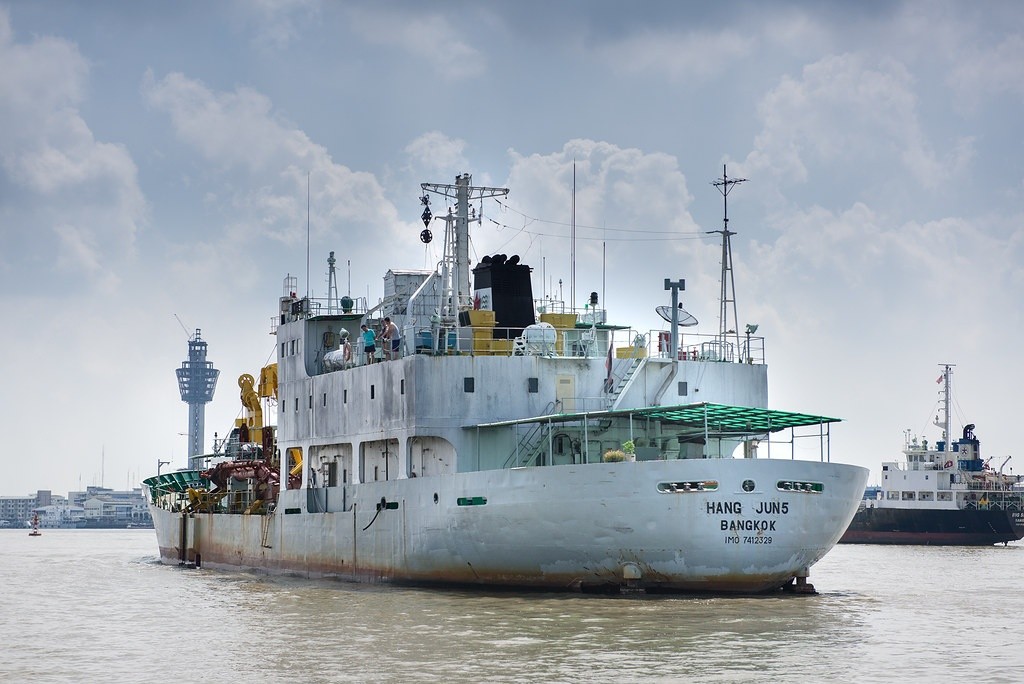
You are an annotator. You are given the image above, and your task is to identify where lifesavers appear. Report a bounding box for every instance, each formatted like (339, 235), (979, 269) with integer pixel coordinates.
(343, 343), (352, 360)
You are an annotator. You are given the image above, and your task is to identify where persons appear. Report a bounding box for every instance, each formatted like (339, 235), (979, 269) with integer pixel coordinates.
(361, 324), (377, 366)
(377, 317), (401, 362)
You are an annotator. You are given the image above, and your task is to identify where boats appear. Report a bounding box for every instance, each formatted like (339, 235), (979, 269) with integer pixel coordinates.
(834, 362), (1024, 547)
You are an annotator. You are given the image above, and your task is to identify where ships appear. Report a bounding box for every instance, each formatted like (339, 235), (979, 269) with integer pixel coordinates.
(142, 153), (872, 599)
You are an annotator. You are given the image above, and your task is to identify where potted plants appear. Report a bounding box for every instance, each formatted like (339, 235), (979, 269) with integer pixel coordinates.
(622, 440), (636, 461)
